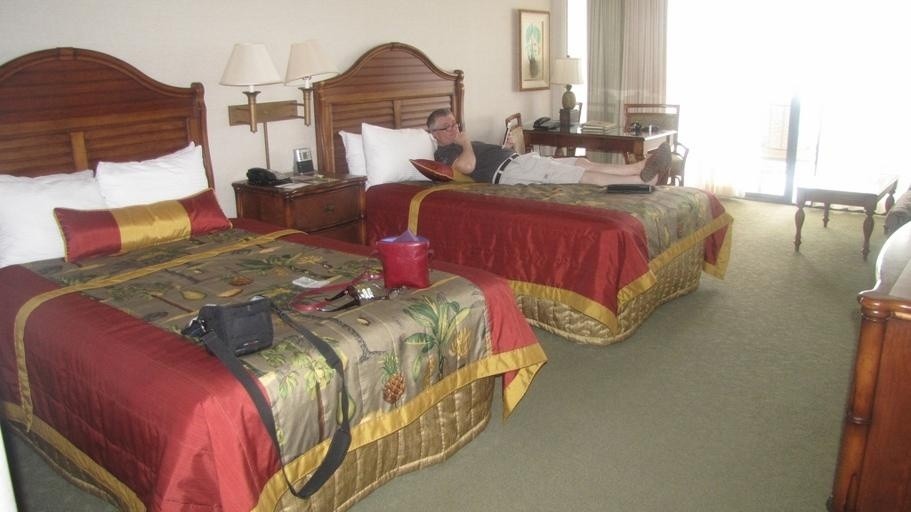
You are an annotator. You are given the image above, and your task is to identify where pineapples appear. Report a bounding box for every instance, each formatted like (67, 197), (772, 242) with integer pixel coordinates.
(376, 348), (406, 404)
(216, 272), (254, 286)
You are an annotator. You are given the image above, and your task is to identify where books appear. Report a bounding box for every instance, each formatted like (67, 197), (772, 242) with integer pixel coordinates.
(579, 119), (616, 134)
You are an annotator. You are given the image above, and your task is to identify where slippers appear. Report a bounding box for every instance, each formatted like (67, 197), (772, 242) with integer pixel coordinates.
(641, 142), (671, 182)
(656, 161), (672, 185)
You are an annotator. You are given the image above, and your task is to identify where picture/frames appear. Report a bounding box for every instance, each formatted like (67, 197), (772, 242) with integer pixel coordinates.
(519, 9), (550, 91)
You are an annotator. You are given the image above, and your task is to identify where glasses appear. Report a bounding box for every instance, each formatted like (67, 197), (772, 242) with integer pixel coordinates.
(434, 121), (460, 132)
(319, 285), (360, 312)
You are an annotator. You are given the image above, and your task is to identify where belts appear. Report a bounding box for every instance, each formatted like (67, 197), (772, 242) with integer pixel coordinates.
(494, 154), (518, 183)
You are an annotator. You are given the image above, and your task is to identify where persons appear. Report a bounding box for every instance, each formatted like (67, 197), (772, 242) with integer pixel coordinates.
(426, 107), (674, 188)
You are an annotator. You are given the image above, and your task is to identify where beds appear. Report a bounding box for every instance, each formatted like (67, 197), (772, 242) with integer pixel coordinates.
(0, 46), (511, 512)
(313, 42), (713, 347)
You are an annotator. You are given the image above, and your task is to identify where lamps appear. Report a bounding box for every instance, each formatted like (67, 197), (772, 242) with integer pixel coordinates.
(551, 54), (583, 109)
(220, 42), (339, 133)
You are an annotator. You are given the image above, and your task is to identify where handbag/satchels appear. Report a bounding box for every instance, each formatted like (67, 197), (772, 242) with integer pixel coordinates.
(197, 298), (274, 356)
(375, 235), (430, 289)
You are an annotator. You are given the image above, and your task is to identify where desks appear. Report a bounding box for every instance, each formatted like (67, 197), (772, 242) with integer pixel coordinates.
(793, 178), (898, 261)
(523, 124), (678, 162)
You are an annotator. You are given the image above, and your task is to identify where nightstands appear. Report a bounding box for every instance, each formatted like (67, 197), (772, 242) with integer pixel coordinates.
(232, 171), (368, 247)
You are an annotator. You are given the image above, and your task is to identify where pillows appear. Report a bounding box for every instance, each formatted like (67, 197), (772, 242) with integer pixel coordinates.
(0, 141), (233, 274)
(338, 123), (475, 193)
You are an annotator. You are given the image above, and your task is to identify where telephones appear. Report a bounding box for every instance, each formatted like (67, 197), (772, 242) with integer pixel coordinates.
(247, 168), (291, 187)
(533, 117), (561, 130)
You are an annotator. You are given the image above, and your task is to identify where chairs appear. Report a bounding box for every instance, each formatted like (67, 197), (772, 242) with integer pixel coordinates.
(622, 104), (689, 186)
(506, 113), (534, 154)
